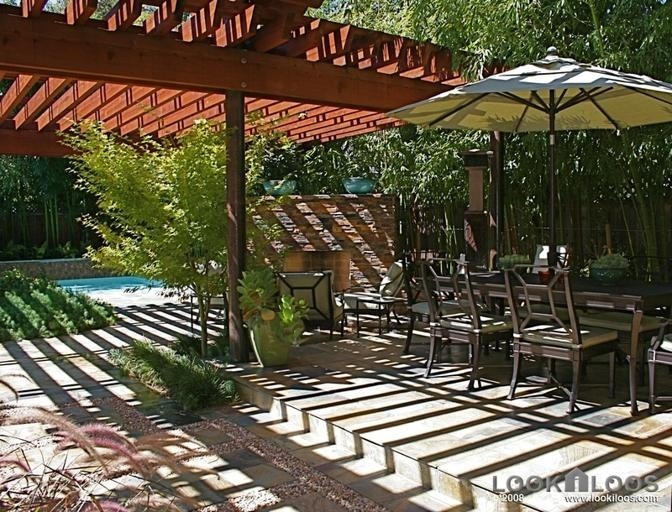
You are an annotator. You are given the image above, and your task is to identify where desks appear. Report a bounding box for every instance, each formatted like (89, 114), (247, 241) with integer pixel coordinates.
(414, 273), (672, 415)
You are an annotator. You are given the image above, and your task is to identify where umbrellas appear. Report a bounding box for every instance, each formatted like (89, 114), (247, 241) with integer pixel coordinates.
(378, 46), (672, 290)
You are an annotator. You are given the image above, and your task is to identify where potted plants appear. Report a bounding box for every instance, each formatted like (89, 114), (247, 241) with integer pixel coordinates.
(236, 268), (309, 367)
(589, 253), (630, 286)
(499, 254), (531, 280)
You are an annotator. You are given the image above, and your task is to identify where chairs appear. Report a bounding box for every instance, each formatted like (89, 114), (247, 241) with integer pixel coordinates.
(579, 256), (672, 386)
(518, 304), (583, 323)
(402, 251), (473, 367)
(532, 244), (570, 274)
(343, 262), (404, 338)
(501, 264), (618, 414)
(647, 315), (672, 414)
(419, 253), (523, 391)
(277, 270), (347, 340)
